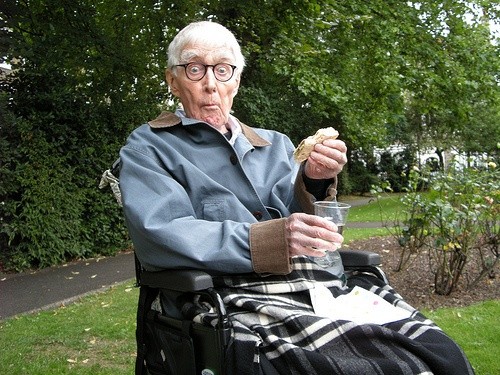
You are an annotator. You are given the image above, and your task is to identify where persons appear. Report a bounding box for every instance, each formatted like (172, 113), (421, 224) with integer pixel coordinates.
(117, 19), (476, 375)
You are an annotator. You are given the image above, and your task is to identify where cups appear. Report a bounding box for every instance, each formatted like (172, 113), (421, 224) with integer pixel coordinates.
(313, 201), (351, 248)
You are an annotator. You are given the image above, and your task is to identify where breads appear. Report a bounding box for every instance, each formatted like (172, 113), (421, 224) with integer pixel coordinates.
(293, 126), (339, 162)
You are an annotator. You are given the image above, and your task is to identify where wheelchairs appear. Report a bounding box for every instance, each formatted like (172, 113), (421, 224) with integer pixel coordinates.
(101, 158), (390, 375)
